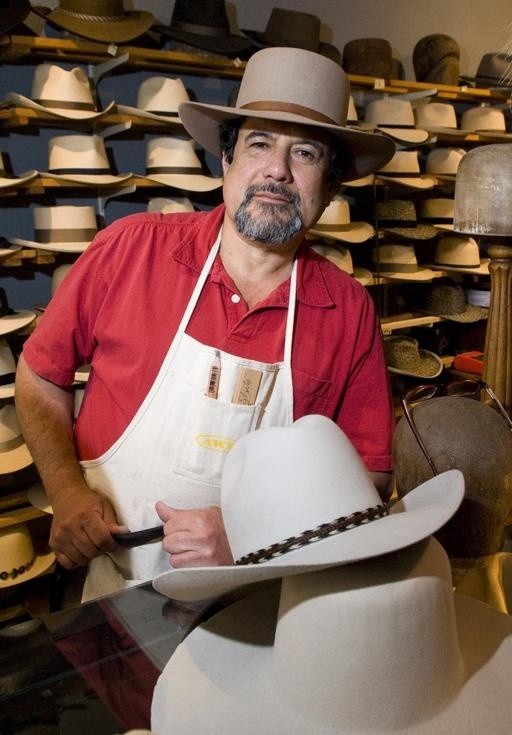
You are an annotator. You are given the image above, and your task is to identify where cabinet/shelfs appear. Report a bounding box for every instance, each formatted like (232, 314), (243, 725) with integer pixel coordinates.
(1, 34), (512, 623)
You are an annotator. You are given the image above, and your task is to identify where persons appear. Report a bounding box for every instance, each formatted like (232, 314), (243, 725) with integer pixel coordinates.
(14, 44), (397, 573)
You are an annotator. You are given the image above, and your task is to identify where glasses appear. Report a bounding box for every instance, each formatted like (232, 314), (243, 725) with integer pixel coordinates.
(402, 380), (512, 477)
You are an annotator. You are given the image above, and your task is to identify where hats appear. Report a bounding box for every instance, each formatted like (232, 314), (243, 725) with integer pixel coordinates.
(308, 94), (512, 322)
(31, 0), (340, 62)
(151, 535), (512, 735)
(459, 53), (512, 87)
(178, 47), (395, 182)
(384, 336), (443, 379)
(0, 264), (93, 588)
(152, 414), (466, 602)
(0, 64), (224, 256)
